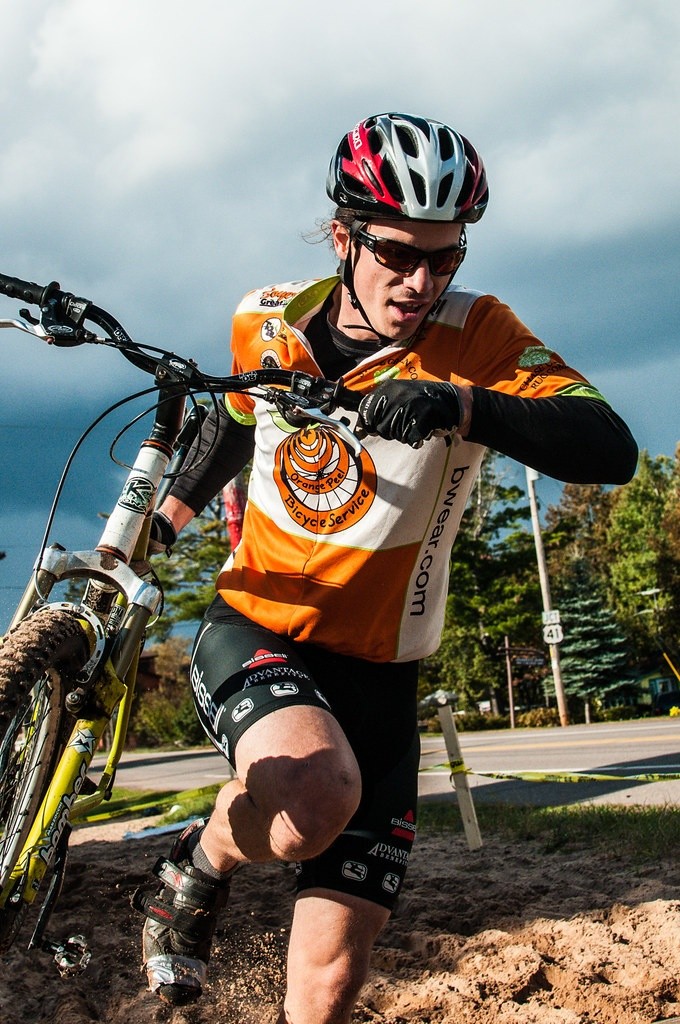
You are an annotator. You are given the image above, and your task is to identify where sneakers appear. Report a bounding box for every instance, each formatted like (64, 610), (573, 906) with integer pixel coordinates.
(142, 817), (234, 1006)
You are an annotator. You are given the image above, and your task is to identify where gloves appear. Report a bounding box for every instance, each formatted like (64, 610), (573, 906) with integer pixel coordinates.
(148, 510), (176, 553)
(352, 378), (464, 450)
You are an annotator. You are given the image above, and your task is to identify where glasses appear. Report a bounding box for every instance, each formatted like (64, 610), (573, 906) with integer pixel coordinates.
(351, 229), (467, 276)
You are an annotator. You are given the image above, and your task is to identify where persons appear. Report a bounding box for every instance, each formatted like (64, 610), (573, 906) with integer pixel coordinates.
(140, 112), (640, 1024)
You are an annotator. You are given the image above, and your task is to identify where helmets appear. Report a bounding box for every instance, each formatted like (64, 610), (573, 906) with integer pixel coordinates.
(325, 113), (489, 223)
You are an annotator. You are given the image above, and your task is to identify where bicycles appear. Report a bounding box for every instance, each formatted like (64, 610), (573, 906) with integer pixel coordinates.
(0, 274), (432, 968)
